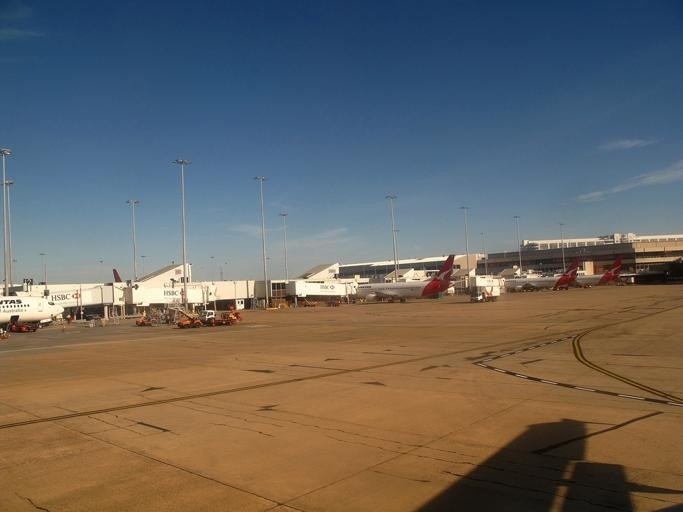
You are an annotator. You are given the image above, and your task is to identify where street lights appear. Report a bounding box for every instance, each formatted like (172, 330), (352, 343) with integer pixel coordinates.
(560, 223), (566, 274)
(0, 149), (13, 295)
(384, 192), (399, 282)
(461, 206), (470, 282)
(127, 199), (139, 281)
(255, 176), (271, 308)
(279, 212), (289, 280)
(480, 232), (487, 275)
(513, 216), (523, 275)
(175, 159), (193, 315)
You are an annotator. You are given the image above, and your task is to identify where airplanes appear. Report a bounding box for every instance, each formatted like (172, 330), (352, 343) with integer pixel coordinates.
(357, 253), (463, 304)
(0, 295), (64, 328)
(504, 263), (578, 291)
(576, 256), (621, 287)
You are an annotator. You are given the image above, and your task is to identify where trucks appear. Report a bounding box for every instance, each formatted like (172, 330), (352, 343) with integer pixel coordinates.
(199, 310), (236, 326)
